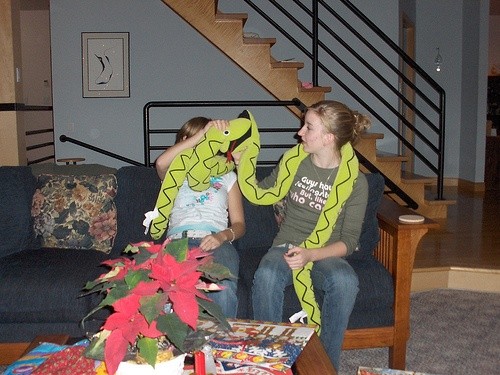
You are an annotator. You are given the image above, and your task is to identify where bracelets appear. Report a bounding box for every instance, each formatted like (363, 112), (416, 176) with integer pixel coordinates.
(225, 228), (235, 245)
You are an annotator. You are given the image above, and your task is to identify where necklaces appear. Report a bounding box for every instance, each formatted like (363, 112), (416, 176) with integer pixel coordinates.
(231, 100), (369, 375)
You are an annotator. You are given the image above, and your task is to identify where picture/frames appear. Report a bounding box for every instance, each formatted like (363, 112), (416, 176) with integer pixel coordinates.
(80, 32), (131, 99)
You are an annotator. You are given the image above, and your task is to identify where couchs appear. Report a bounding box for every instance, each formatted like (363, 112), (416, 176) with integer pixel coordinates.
(0, 164), (440, 370)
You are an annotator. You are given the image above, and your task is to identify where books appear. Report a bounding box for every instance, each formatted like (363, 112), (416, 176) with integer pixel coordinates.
(174, 312), (319, 375)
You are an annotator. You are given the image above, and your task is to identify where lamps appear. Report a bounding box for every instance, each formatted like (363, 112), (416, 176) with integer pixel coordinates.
(434, 48), (443, 72)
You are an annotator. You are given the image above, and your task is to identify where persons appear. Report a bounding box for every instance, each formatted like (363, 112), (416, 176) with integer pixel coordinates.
(156, 117), (246, 318)
(311, 154), (341, 189)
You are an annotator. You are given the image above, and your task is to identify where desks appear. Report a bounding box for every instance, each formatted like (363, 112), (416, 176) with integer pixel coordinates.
(0, 331), (339, 374)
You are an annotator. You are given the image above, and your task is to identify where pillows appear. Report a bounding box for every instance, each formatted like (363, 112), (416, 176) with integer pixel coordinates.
(31, 175), (118, 256)
